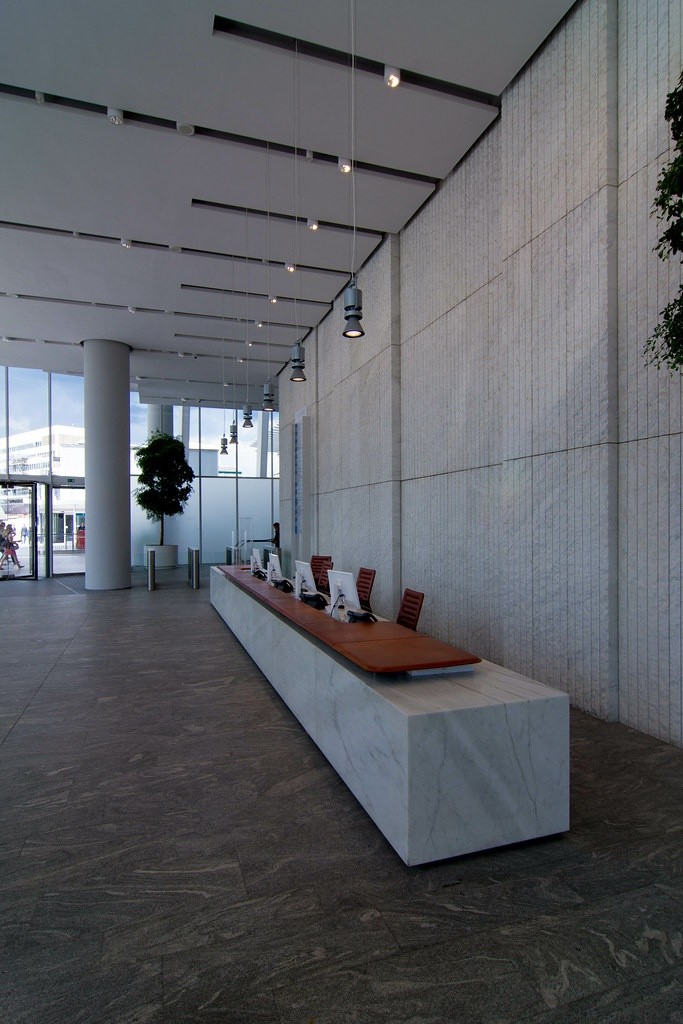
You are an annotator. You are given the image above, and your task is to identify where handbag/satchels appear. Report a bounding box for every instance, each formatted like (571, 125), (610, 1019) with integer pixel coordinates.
(4, 535), (11, 549)
(10, 540), (19, 550)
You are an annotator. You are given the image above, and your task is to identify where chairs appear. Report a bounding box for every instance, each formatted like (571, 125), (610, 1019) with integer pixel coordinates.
(317, 560), (334, 591)
(395, 588), (424, 630)
(309, 555), (331, 580)
(356, 567), (376, 602)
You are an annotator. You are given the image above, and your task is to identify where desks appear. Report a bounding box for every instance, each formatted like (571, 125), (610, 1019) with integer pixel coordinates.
(208, 559), (571, 868)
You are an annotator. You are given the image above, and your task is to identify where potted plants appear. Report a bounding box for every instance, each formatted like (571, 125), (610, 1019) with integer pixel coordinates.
(132, 429), (196, 570)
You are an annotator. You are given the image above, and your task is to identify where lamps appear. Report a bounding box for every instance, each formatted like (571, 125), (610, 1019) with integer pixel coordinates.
(230, 256), (239, 445)
(219, 317), (229, 457)
(342, 1), (365, 341)
(263, 141), (274, 415)
(291, 40), (308, 384)
(242, 207), (255, 429)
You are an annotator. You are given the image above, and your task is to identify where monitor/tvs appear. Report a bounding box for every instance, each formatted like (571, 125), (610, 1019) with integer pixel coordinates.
(251, 548), (362, 619)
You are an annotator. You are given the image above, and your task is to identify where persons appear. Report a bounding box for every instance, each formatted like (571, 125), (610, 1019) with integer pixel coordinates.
(0, 522), (26, 571)
(273, 522), (280, 554)
(78, 522), (85, 530)
